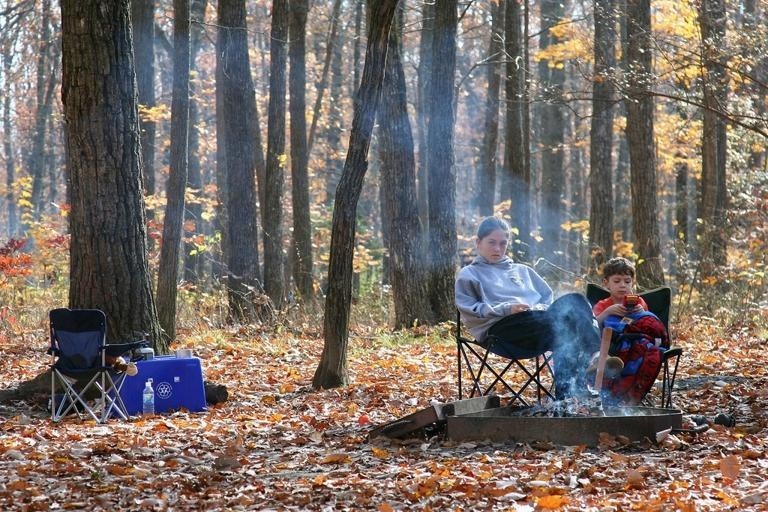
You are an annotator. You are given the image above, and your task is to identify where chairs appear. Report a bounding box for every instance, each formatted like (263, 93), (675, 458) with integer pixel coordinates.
(585, 282), (682, 409)
(456, 307), (556, 407)
(46, 308), (132, 426)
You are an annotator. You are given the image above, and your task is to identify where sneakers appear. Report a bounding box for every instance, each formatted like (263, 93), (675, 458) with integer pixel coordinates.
(583, 356), (625, 382)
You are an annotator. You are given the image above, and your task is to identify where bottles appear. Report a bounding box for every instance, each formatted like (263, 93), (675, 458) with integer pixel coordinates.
(143, 382), (154, 416)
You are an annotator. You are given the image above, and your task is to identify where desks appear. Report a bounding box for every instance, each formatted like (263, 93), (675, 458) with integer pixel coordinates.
(108, 355), (206, 418)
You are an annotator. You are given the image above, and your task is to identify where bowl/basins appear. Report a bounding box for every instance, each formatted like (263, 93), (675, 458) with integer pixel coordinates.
(174, 349), (192, 358)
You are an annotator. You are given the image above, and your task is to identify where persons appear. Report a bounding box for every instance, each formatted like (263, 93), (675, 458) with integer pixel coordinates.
(589, 255), (670, 405)
(455, 215), (624, 406)
